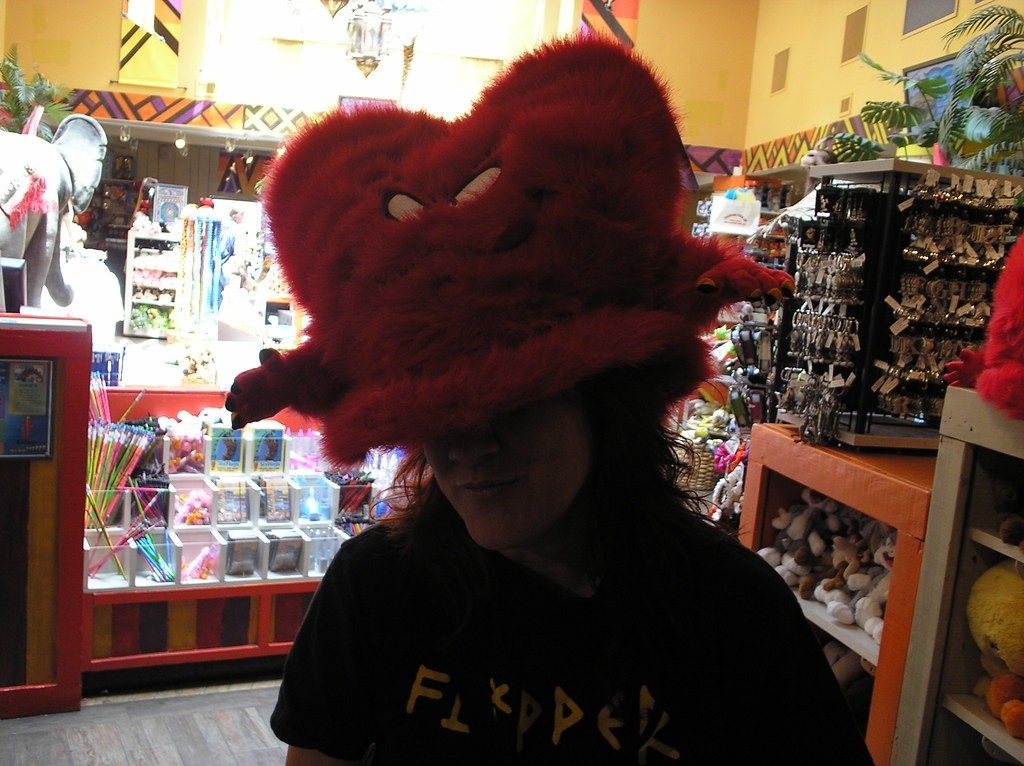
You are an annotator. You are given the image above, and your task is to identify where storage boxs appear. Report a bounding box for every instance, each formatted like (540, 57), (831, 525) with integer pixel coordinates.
(84, 433), (388, 593)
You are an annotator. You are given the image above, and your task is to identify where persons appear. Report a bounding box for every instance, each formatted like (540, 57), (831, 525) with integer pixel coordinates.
(224, 33), (876, 766)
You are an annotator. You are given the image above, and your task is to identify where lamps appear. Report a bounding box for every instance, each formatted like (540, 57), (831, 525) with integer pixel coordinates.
(175, 129), (190, 158)
(119, 124), (131, 142)
(243, 149), (254, 164)
(224, 137), (236, 152)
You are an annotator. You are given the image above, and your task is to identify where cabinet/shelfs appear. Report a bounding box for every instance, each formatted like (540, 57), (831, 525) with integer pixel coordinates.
(683, 158), (1024, 766)
(123, 230), (181, 339)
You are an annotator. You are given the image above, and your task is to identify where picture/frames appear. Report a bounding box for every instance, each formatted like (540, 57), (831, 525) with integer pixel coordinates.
(902, 50), (980, 135)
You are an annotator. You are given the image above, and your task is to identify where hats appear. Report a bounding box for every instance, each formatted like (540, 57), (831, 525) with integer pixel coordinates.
(224, 33), (793, 472)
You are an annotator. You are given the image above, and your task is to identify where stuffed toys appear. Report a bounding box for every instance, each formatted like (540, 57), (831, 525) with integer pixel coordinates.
(987, 458), (1024, 545)
(966, 558), (1024, 739)
(943, 232), (1024, 421)
(756, 487), (897, 646)
